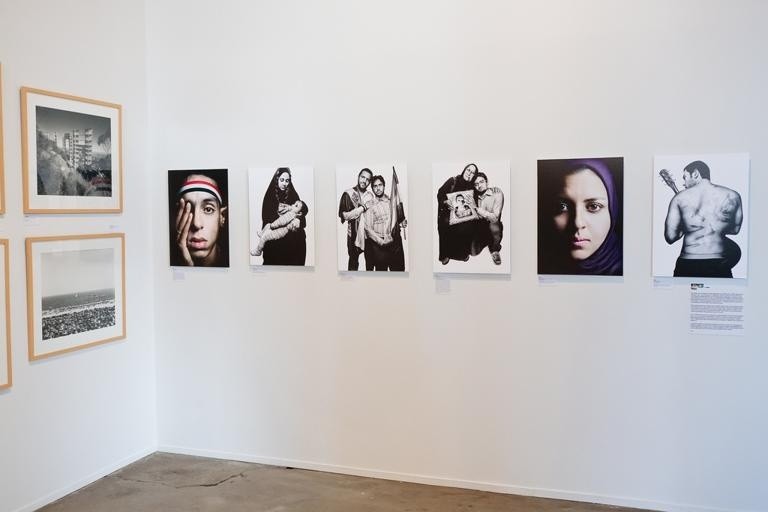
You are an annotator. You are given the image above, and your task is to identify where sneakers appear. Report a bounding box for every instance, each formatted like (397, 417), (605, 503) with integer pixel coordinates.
(443, 256), (449, 264)
(492, 252), (501, 265)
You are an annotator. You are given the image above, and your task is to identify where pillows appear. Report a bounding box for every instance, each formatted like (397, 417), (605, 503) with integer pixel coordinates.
(446, 190), (482, 225)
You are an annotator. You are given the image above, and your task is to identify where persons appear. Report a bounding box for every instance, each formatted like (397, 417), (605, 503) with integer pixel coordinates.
(339, 167), (375, 270)
(249, 199), (308, 257)
(466, 171), (505, 266)
(353, 174), (407, 272)
(436, 163), (503, 264)
(452, 193), (473, 219)
(545, 160), (625, 275)
(665, 160), (745, 278)
(171, 173), (226, 266)
(261, 168), (307, 266)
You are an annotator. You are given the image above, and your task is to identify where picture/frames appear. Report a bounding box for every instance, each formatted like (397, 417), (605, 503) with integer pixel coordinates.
(0, 62), (6, 215)
(24, 231), (127, 363)
(20, 85), (124, 215)
(0, 238), (12, 391)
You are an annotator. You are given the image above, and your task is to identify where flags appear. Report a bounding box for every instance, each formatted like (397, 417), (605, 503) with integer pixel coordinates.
(390, 168), (407, 243)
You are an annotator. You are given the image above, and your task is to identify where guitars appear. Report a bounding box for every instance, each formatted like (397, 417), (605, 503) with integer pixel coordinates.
(660, 168), (741, 271)
